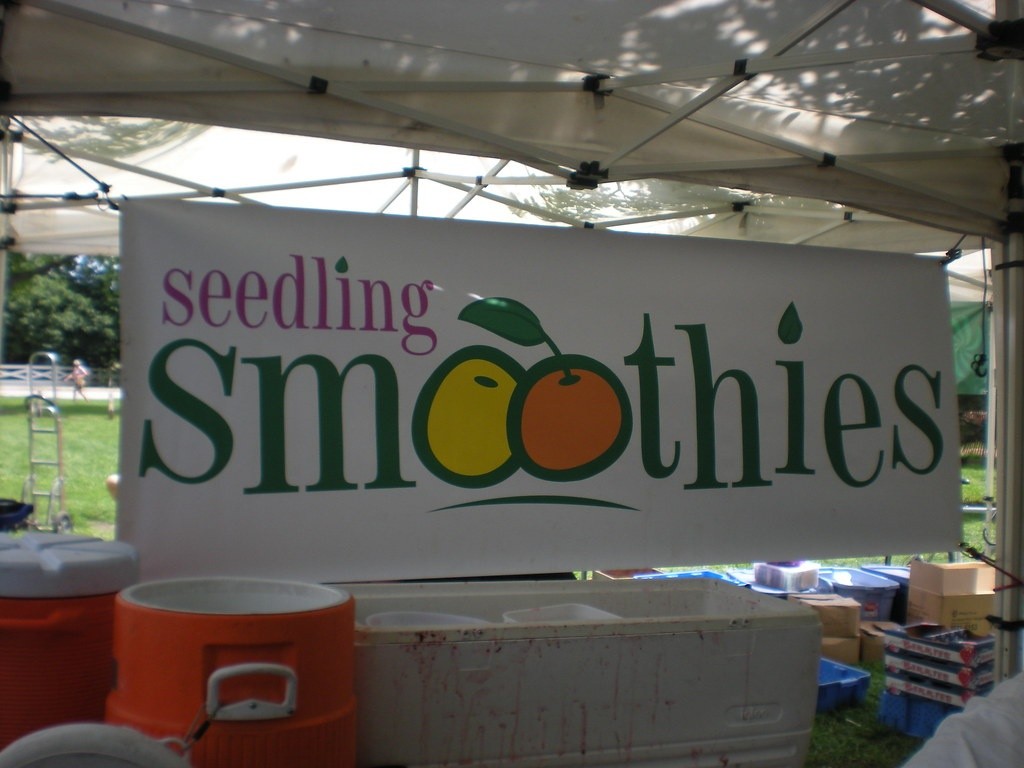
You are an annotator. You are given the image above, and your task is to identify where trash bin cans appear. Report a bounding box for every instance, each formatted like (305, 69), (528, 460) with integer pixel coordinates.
(0, 498), (33, 531)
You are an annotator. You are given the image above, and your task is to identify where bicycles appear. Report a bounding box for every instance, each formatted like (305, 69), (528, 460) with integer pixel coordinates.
(885, 479), (997, 568)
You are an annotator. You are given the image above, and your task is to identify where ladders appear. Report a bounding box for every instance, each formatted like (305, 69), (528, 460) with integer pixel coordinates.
(22, 351), (75, 538)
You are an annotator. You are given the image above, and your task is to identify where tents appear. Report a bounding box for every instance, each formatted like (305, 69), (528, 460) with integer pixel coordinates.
(0, 0), (1023, 683)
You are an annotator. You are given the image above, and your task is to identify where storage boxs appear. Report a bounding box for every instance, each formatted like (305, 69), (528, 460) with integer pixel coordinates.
(592, 562), (997, 742)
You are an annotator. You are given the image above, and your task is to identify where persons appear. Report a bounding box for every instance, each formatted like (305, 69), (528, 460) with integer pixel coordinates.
(64, 360), (88, 402)
